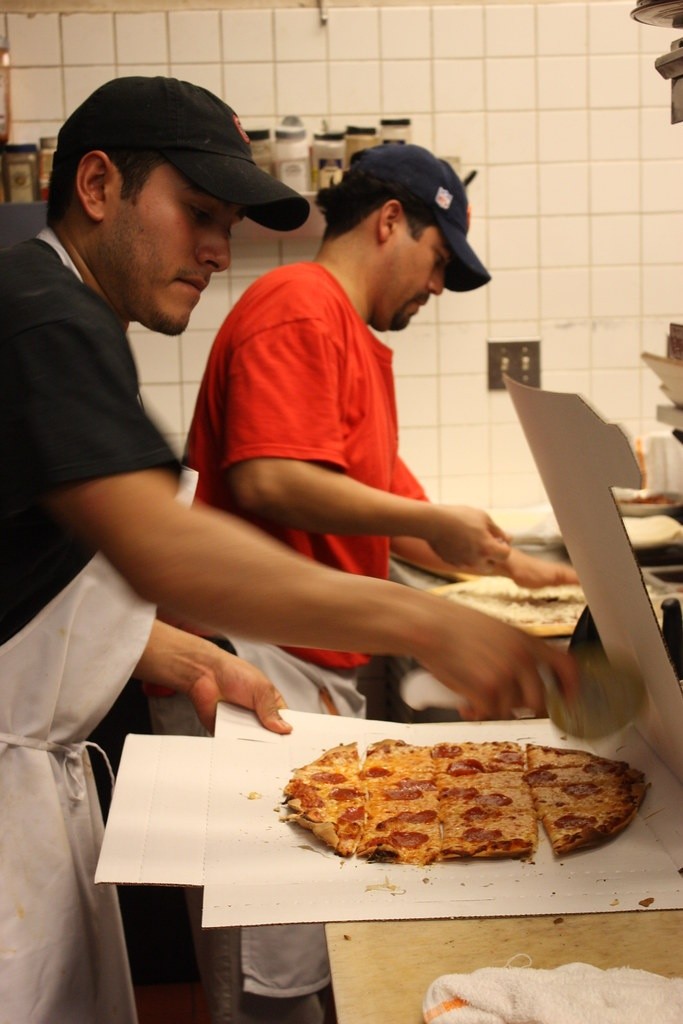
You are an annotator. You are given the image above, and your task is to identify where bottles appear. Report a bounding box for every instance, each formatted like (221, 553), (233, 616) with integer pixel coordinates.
(243, 118), (412, 192)
(0, 137), (58, 204)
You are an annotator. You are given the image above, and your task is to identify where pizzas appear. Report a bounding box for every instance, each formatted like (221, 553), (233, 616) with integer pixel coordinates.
(276, 739), (647, 864)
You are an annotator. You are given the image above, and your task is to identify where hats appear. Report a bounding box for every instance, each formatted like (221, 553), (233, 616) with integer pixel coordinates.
(347, 142), (491, 292)
(58, 76), (310, 231)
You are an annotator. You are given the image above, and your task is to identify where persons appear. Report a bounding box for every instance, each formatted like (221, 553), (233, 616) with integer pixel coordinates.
(149, 143), (578, 1023)
(0, 76), (579, 1024)
